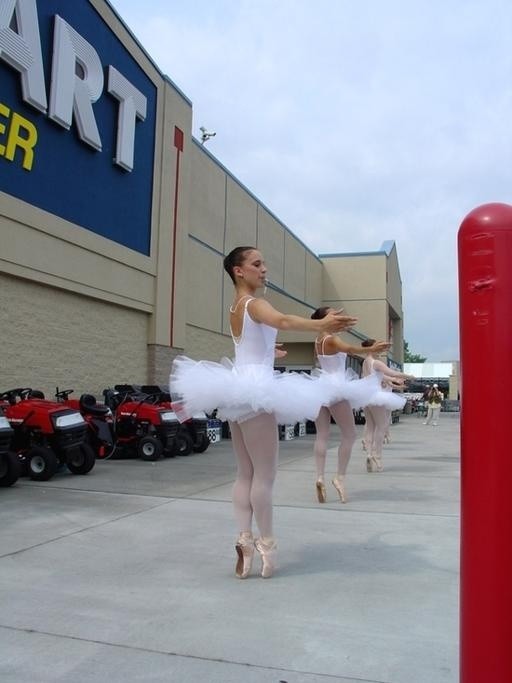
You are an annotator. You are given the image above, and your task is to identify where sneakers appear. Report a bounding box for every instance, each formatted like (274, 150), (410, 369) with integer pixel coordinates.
(362, 439), (368, 451)
(316, 475), (326, 503)
(366, 454), (383, 472)
(384, 432), (390, 444)
(332, 475), (347, 503)
(235, 531), (277, 578)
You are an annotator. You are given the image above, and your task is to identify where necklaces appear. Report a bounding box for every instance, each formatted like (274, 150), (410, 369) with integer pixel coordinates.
(229, 294), (254, 314)
(315, 334), (330, 344)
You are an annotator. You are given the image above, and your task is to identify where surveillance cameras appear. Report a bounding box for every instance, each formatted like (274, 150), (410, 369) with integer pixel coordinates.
(199, 125), (217, 140)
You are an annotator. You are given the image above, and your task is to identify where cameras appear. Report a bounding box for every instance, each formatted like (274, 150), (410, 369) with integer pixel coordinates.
(433, 387), (435, 390)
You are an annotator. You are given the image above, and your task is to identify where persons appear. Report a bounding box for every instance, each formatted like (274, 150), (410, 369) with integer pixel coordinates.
(309, 306), (394, 502)
(419, 384), (443, 426)
(361, 337), (408, 473)
(168, 244), (359, 579)
